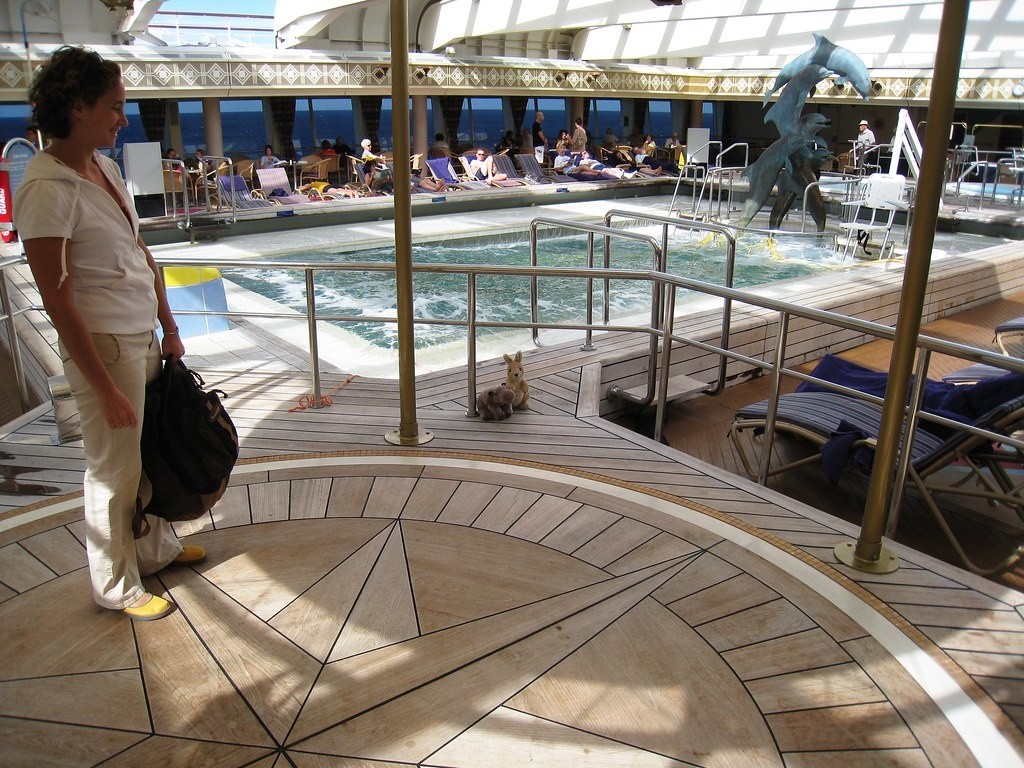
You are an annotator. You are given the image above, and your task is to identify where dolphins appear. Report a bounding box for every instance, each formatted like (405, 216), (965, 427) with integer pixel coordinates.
(785, 113), (831, 174)
(761, 32), (872, 101)
(764, 64), (835, 241)
(812, 149), (833, 170)
(735, 134), (811, 238)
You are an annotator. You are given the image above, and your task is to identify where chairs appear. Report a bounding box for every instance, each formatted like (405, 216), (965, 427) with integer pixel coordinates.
(354, 162), (437, 198)
(994, 315), (1024, 356)
(646, 145), (657, 157)
(429, 147), (465, 174)
(423, 156), (493, 190)
(820, 153), (1020, 184)
(514, 154), (577, 183)
(410, 153), (424, 170)
(607, 152), (662, 178)
(544, 149), (610, 182)
(345, 155), (365, 183)
(573, 153), (628, 179)
(941, 362), (1013, 385)
(492, 154), (541, 186)
(458, 155), (522, 189)
(657, 146), (671, 162)
(730, 355), (1024, 576)
(519, 144), (553, 168)
(163, 152), (365, 211)
(593, 144), (613, 163)
(497, 148), (510, 155)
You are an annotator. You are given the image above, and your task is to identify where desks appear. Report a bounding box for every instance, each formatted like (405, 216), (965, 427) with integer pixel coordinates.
(279, 163), (307, 190)
(662, 147), (675, 163)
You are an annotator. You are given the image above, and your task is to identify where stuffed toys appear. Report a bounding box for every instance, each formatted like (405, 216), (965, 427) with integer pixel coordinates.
(476, 350), (530, 422)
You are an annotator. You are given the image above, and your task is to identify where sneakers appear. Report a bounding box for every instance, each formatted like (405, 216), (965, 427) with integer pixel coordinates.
(121, 591), (171, 621)
(172, 546), (206, 563)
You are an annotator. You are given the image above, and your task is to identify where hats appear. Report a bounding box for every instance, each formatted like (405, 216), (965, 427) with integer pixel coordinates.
(857, 120), (870, 128)
(360, 139), (371, 148)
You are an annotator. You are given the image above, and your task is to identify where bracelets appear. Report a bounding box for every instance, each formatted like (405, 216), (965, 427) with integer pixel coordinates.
(163, 326), (179, 334)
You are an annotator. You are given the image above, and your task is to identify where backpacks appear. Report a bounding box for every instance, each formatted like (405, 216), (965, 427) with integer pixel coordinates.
(141, 350), (240, 521)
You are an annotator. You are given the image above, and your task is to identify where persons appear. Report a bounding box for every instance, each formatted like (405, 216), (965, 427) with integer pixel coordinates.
(321, 137), (354, 180)
(10, 44), (215, 620)
(164, 148), (211, 172)
(520, 112), (547, 167)
(360, 132), (452, 194)
(554, 117), (685, 179)
(298, 182), (359, 199)
(260, 145), (281, 168)
(470, 128), (518, 185)
(857, 119), (876, 175)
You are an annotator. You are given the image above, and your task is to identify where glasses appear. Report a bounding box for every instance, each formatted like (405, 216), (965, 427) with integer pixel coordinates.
(366, 144), (372, 147)
(478, 154), (485, 156)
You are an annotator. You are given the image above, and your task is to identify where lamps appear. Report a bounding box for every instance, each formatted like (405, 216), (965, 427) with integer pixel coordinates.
(416, 68), (430, 80)
(556, 72), (569, 83)
(871, 80), (882, 91)
(375, 67), (389, 79)
(586, 74), (599, 84)
(832, 79), (844, 90)
